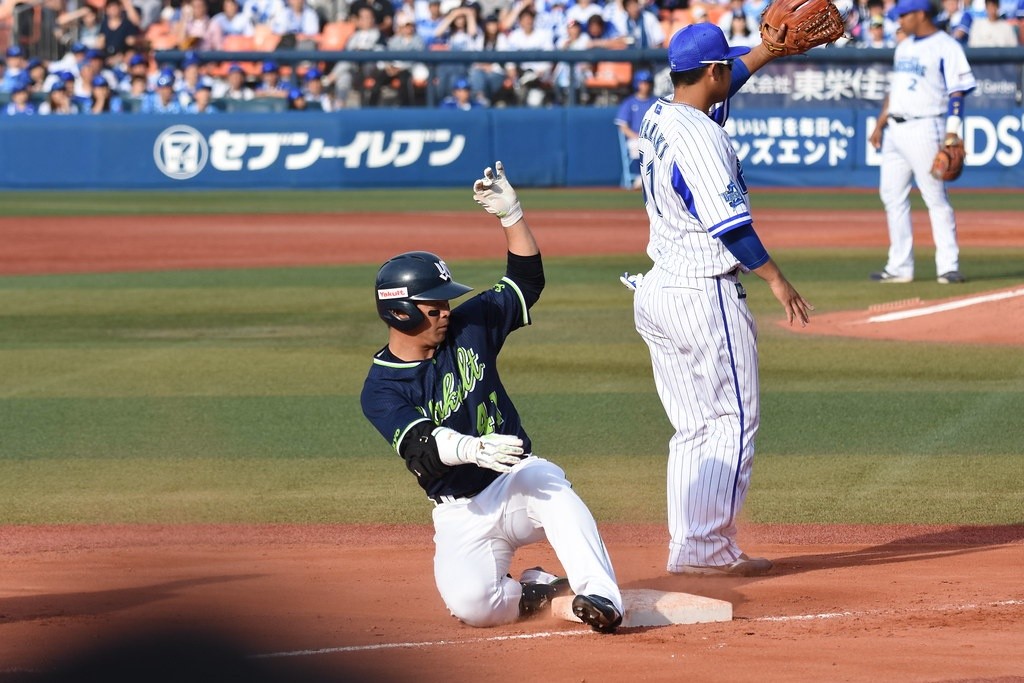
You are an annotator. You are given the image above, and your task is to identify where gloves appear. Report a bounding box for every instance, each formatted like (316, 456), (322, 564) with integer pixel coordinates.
(620, 270), (646, 292)
(459, 435), (524, 474)
(473, 161), (523, 227)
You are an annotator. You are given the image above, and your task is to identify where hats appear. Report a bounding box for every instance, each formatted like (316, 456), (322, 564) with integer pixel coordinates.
(667, 22), (751, 72)
(7, 41), (322, 102)
(888, 0), (932, 18)
(451, 81), (472, 90)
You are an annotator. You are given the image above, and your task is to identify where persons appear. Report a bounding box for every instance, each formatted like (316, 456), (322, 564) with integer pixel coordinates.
(868, 0), (978, 285)
(0, 0), (1024, 106)
(633, 22), (817, 576)
(612, 69), (662, 190)
(357, 162), (626, 634)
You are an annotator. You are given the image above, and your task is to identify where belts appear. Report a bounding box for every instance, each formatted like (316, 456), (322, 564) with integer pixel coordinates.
(893, 116), (905, 124)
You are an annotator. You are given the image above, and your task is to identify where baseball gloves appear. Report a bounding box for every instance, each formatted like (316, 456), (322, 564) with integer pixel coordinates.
(758, 0), (858, 59)
(929, 137), (967, 182)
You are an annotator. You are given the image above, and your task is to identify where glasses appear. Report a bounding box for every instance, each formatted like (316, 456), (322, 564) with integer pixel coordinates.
(698, 58), (735, 72)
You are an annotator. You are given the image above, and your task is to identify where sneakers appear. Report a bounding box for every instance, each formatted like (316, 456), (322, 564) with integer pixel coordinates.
(870, 268), (913, 284)
(937, 273), (966, 285)
(573, 595), (623, 633)
(520, 568), (572, 617)
(669, 552), (772, 579)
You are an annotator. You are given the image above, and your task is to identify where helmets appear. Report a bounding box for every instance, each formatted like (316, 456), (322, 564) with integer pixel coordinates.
(634, 69), (654, 92)
(376, 250), (476, 333)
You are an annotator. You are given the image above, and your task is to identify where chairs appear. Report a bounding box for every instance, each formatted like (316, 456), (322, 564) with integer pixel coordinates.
(586, 37), (632, 107)
